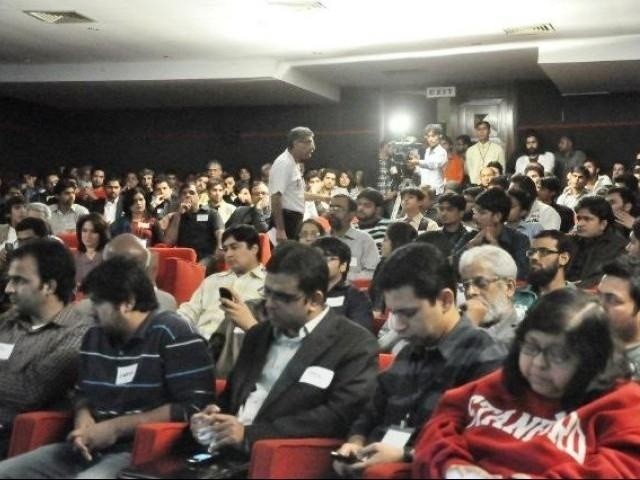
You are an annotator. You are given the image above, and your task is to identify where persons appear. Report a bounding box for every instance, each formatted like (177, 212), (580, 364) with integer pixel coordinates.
(168, 239), (381, 465)
(0, 256), (216, 480)
(0, 237), (95, 435)
(1, 121), (639, 382)
(412, 287), (640, 479)
(323, 241), (502, 480)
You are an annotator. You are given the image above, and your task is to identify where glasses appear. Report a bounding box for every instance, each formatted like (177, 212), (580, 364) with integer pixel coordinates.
(526, 249), (560, 259)
(456, 277), (500, 293)
(326, 207), (349, 213)
(257, 284), (305, 305)
